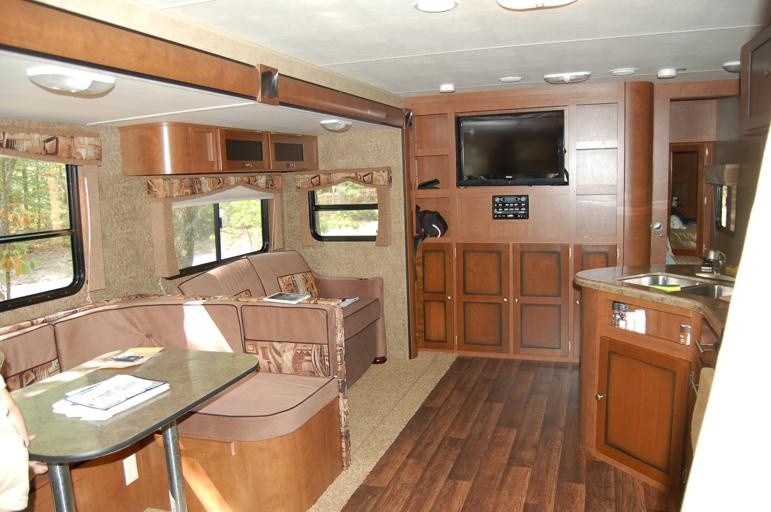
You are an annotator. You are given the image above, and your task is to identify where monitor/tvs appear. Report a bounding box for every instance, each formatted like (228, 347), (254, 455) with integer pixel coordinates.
(454, 110), (565, 187)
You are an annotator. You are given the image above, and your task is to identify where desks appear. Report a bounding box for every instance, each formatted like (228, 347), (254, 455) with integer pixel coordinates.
(8, 345), (262, 511)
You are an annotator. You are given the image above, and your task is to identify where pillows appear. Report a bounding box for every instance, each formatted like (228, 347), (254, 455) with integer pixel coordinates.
(276, 272), (320, 298)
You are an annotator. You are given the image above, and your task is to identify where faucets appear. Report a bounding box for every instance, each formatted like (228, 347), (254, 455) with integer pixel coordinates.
(706, 248), (727, 273)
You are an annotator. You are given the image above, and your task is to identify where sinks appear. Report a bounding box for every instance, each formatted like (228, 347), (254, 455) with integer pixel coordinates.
(677, 283), (734, 299)
(614, 272), (714, 292)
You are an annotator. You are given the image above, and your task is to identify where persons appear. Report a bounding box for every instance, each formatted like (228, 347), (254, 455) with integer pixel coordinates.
(0, 349), (50, 510)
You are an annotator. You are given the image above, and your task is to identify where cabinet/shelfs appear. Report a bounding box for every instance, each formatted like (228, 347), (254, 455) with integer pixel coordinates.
(453, 242), (570, 361)
(574, 242), (616, 358)
(216, 125), (317, 173)
(416, 242), (453, 354)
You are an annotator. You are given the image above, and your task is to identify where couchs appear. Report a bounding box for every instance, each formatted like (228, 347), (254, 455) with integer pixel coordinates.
(164, 249), (385, 388)
(0, 294), (349, 511)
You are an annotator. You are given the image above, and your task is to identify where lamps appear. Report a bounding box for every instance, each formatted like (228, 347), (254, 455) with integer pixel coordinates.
(24, 64), (114, 97)
(543, 71), (590, 84)
(320, 119), (352, 135)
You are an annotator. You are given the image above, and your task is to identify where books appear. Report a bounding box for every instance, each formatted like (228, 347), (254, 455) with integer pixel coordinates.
(63, 374), (170, 418)
